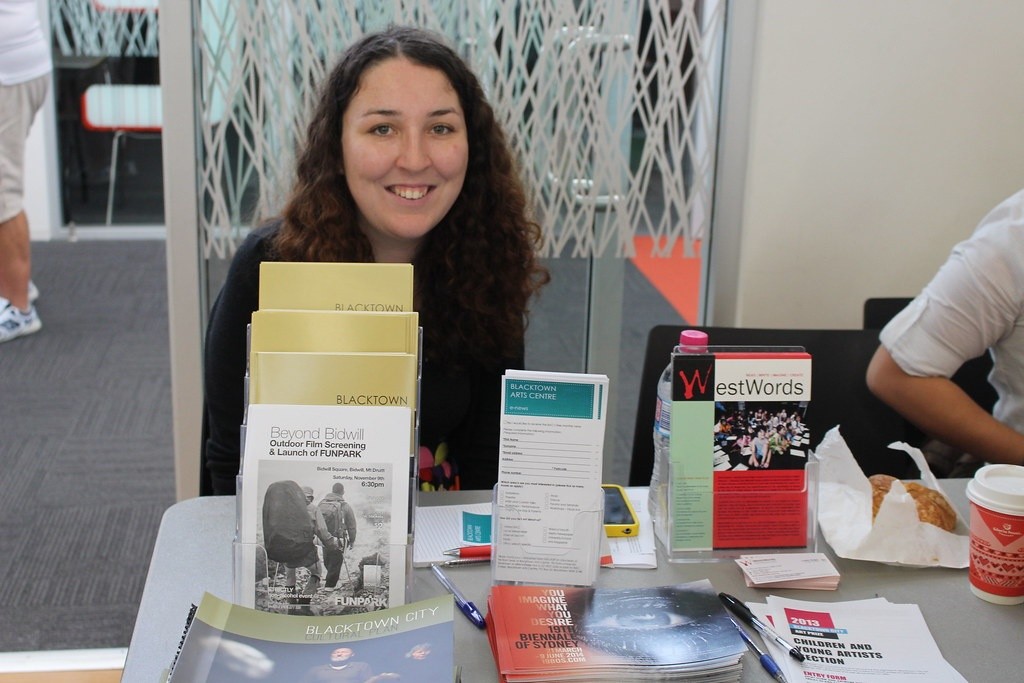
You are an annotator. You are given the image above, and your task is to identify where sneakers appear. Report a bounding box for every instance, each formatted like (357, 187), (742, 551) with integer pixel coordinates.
(0, 279), (40, 312)
(0, 303), (41, 342)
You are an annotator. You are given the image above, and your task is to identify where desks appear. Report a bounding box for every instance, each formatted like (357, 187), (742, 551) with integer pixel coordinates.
(118, 477), (1024, 683)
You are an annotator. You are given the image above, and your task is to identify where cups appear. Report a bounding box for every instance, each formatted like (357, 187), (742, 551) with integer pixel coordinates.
(965, 463), (1024, 606)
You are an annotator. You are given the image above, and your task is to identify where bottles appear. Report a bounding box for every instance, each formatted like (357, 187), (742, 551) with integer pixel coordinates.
(646, 330), (709, 522)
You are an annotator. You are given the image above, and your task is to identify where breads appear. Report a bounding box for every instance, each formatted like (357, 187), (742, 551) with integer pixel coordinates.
(867, 473), (957, 533)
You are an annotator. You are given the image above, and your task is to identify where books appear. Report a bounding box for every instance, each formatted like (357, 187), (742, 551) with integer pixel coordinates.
(165, 259), (455, 616)
(483, 576), (749, 683)
(168, 590), (462, 683)
(667, 336), (812, 553)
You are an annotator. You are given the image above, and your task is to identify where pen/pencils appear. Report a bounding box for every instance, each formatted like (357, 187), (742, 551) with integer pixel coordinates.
(430, 563), (485, 628)
(718, 592), (806, 662)
(443, 544), (491, 556)
(727, 613), (787, 683)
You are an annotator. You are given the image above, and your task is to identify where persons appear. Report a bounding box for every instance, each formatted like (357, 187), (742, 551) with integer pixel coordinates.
(317, 482), (358, 592)
(281, 487), (346, 615)
(302, 642), (432, 683)
(197, 24), (551, 498)
(715, 406), (803, 468)
(866, 188), (1024, 466)
(0, 0), (53, 342)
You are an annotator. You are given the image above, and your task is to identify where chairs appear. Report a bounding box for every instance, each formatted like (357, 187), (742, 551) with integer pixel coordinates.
(627, 297), (1000, 485)
(81, 0), (246, 227)
